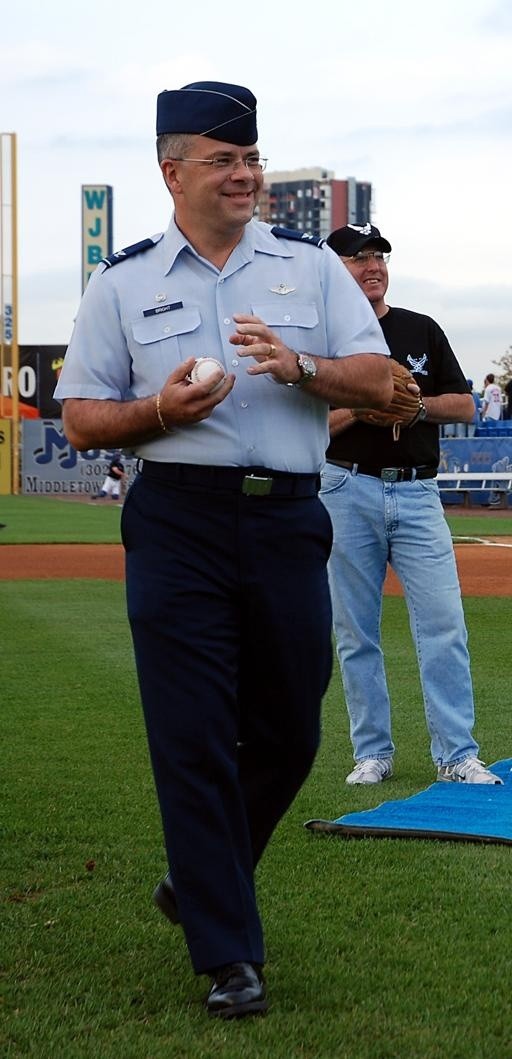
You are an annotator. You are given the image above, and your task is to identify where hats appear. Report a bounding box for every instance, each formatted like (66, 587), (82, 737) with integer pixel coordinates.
(154, 79), (261, 148)
(327, 221), (395, 259)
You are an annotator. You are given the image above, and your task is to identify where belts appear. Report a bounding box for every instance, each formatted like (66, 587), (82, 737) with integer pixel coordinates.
(135, 461), (322, 499)
(325, 456), (440, 483)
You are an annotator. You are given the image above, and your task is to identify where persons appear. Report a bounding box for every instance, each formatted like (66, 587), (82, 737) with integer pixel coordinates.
(316, 223), (504, 787)
(457, 377), (481, 438)
(90, 449), (126, 499)
(50, 80), (397, 1020)
(480, 372), (502, 421)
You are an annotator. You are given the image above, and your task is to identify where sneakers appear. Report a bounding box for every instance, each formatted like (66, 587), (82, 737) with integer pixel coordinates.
(149, 869), (188, 927)
(343, 754), (401, 785)
(430, 752), (493, 788)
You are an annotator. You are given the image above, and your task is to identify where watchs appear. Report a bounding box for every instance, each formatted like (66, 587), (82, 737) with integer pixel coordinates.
(417, 400), (428, 420)
(283, 346), (318, 387)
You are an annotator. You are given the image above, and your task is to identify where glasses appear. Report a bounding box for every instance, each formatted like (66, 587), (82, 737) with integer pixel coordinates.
(168, 153), (267, 173)
(343, 251), (391, 264)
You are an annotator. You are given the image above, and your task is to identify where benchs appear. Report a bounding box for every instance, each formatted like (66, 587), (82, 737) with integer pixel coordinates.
(433, 471), (512, 509)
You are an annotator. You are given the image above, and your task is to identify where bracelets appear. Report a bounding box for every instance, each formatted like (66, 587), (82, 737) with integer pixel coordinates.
(152, 392), (178, 437)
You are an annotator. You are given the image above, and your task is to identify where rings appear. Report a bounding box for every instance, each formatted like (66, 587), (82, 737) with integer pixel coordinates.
(269, 343), (278, 358)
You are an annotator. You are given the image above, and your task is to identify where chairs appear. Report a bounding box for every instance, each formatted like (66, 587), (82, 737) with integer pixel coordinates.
(474, 416), (511, 437)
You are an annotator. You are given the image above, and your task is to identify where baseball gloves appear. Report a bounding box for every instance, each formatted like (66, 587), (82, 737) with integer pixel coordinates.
(352, 358), (423, 427)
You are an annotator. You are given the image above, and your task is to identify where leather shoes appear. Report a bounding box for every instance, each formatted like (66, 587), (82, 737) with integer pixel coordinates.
(201, 960), (273, 1021)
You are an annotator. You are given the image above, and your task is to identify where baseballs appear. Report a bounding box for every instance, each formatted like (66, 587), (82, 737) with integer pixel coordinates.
(190, 354), (229, 398)
